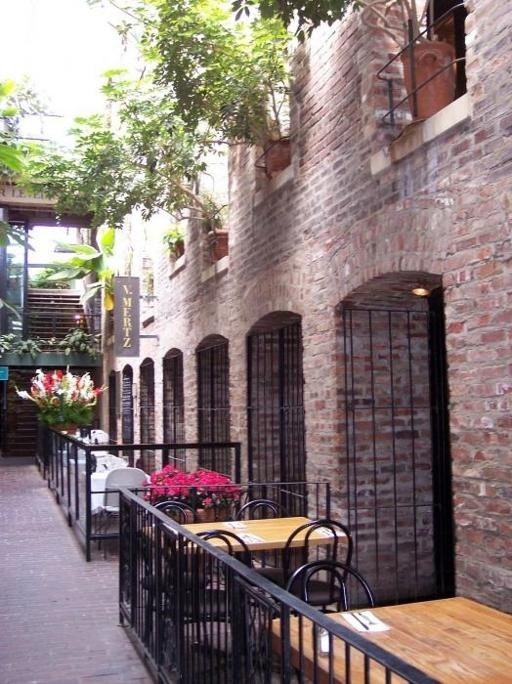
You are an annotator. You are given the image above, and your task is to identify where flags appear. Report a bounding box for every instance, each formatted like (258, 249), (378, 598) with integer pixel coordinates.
(113, 275), (140, 357)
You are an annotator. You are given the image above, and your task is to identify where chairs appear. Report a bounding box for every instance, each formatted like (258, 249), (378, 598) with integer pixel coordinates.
(148, 494), (354, 681)
(98, 467), (149, 561)
(282, 561), (373, 615)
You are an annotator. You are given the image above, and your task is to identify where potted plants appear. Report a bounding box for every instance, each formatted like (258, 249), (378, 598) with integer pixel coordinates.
(162, 229), (184, 258)
(248, 16), (291, 173)
(198, 198), (228, 261)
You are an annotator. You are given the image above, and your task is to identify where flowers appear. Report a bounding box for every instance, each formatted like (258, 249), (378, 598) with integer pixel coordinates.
(142, 464), (243, 508)
(16, 367), (111, 425)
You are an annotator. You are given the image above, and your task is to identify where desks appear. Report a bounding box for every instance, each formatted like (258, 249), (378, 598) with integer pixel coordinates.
(262, 595), (511, 683)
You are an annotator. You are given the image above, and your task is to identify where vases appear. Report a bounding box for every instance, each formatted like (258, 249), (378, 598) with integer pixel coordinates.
(398, 40), (455, 123)
(49, 422), (78, 434)
